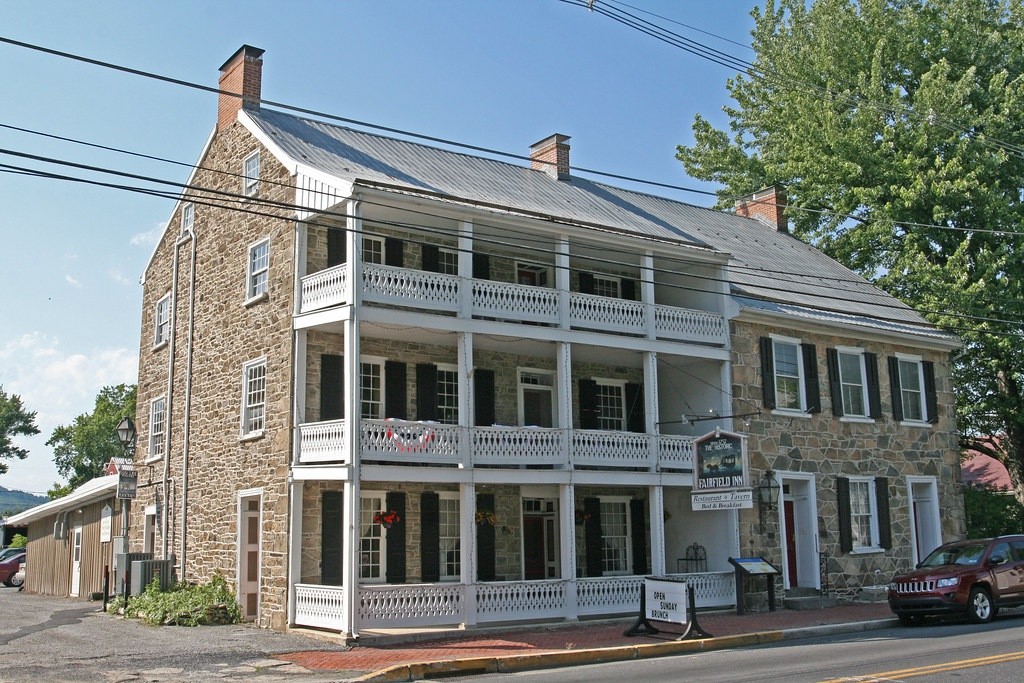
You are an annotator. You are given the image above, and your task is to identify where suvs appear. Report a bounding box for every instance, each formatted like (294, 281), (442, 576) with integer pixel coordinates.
(888, 534), (1024, 627)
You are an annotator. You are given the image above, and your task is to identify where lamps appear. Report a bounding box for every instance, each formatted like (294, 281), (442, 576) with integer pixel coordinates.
(753, 467), (780, 536)
(115, 416), (154, 483)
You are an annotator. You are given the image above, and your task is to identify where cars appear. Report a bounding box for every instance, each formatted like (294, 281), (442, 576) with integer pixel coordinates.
(0, 548), (26, 562)
(0, 553), (26, 587)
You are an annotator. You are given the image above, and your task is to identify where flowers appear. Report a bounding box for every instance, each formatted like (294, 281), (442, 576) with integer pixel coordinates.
(373, 510), (400, 526)
(475, 512), (496, 526)
